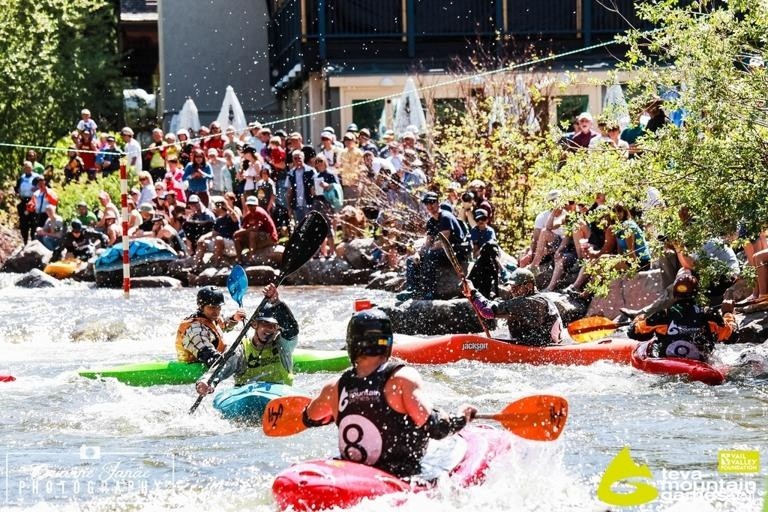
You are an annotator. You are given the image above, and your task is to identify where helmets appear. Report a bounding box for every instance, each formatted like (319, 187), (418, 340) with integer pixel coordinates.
(252, 303), (280, 324)
(673, 275), (698, 298)
(346, 309), (394, 359)
(70, 221), (82, 232)
(503, 269), (535, 286)
(196, 285), (226, 307)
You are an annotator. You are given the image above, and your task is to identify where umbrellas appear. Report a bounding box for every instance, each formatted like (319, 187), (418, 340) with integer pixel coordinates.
(217, 85), (247, 129)
(604, 74), (629, 128)
(179, 97), (199, 132)
(515, 75), (541, 131)
(398, 78), (426, 134)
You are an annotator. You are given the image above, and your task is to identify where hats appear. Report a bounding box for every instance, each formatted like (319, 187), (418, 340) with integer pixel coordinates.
(578, 111), (594, 122)
(75, 108), (493, 221)
(546, 190), (561, 201)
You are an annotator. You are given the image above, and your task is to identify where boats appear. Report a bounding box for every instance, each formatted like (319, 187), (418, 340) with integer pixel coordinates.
(45, 236), (179, 279)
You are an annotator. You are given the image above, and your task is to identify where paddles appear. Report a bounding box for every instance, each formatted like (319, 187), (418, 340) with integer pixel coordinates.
(570, 292), (768, 343)
(186, 211), (330, 413)
(227, 264), (247, 342)
(262, 396), (568, 439)
(438, 234), (493, 338)
